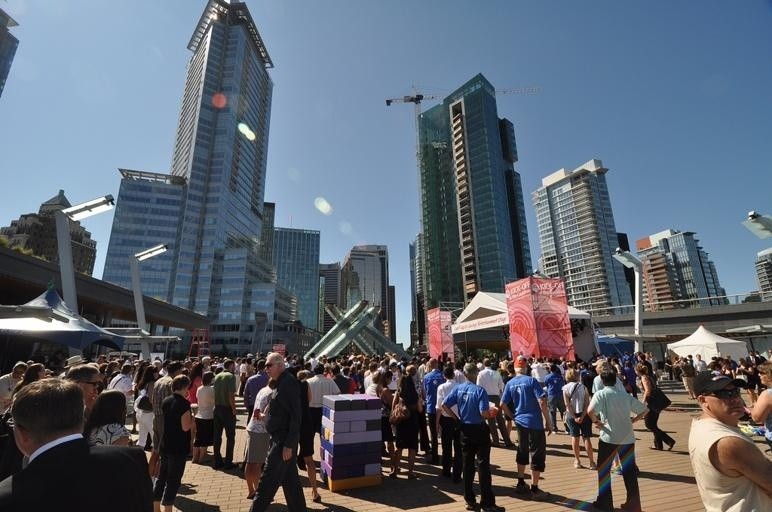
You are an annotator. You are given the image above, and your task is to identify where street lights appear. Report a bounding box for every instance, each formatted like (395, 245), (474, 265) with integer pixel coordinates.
(612, 246), (644, 357)
(742, 209), (772, 241)
(128, 244), (170, 364)
(54, 193), (115, 360)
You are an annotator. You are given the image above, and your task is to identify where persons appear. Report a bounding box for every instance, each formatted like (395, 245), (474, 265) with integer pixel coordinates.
(5, 328), (770, 510)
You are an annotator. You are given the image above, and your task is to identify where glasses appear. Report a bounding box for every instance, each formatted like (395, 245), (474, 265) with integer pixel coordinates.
(7, 417), (30, 432)
(703, 389), (740, 399)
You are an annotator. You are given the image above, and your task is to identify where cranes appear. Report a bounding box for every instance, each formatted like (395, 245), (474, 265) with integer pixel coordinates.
(385, 81), (543, 335)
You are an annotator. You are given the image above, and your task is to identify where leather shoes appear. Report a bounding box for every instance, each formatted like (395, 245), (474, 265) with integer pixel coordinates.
(313, 496), (321, 503)
(668, 440), (675, 450)
(247, 493), (255, 499)
(648, 447), (663, 450)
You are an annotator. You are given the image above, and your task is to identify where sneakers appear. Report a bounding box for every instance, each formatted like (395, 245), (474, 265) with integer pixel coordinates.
(610, 466), (622, 475)
(529, 490), (550, 500)
(575, 463), (582, 469)
(590, 462), (597, 470)
(466, 503), (505, 512)
(516, 484), (530, 495)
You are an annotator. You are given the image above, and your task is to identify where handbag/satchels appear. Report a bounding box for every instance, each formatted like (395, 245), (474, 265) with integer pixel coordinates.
(646, 388), (671, 414)
(736, 375), (748, 383)
(137, 396), (153, 411)
(389, 397), (409, 424)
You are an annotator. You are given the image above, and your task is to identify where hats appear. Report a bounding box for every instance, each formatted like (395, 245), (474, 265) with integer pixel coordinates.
(465, 363), (475, 371)
(514, 360), (527, 368)
(64, 355), (88, 369)
(592, 359), (604, 366)
(692, 370), (734, 392)
